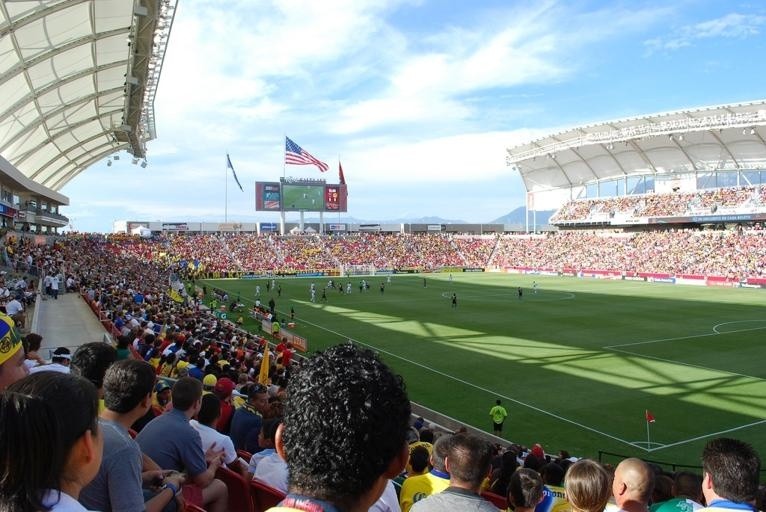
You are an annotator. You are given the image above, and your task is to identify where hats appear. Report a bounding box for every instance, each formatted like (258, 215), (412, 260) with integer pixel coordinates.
(177, 360), (236, 396)
(156, 380), (173, 390)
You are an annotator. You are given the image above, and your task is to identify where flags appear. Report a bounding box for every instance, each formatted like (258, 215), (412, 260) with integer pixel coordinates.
(224, 150), (245, 193)
(337, 162), (351, 194)
(285, 136), (330, 176)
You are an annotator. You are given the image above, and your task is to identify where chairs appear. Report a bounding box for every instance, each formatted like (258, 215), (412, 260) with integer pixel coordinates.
(77, 284), (511, 512)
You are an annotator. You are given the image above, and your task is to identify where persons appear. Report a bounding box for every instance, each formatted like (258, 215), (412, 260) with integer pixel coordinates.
(486, 397), (509, 436)
(550, 184), (766, 226)
(1, 315), (307, 511)
(277, 416), (765, 511)
(0, 228), (766, 314)
(262, 339), (413, 510)
